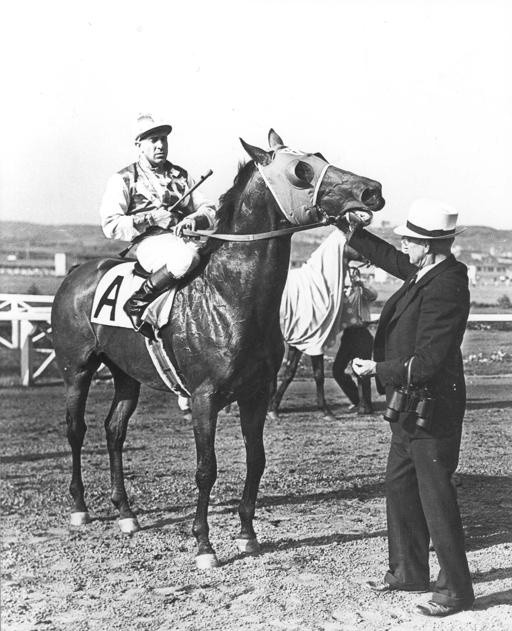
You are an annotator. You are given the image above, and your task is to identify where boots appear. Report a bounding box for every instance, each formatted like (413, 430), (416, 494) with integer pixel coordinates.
(121, 263), (180, 340)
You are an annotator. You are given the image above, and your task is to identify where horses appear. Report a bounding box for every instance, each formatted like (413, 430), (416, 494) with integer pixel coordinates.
(50, 128), (386, 570)
(178, 244), (367, 423)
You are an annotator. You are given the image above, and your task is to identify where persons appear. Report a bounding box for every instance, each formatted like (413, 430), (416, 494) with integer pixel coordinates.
(337, 201), (474, 617)
(331, 268), (380, 414)
(99, 115), (221, 337)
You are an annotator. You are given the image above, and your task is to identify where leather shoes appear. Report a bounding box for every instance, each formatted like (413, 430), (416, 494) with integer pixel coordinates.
(363, 578), (399, 594)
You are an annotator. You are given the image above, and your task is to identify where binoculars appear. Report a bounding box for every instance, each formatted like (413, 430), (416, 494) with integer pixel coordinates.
(384, 383), (437, 431)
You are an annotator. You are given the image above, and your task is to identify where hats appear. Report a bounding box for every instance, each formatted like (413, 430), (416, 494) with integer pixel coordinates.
(132, 114), (174, 143)
(393, 205), (468, 243)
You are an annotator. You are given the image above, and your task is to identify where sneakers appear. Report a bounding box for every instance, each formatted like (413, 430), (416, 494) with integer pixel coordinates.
(348, 400), (373, 415)
(415, 597), (464, 618)
